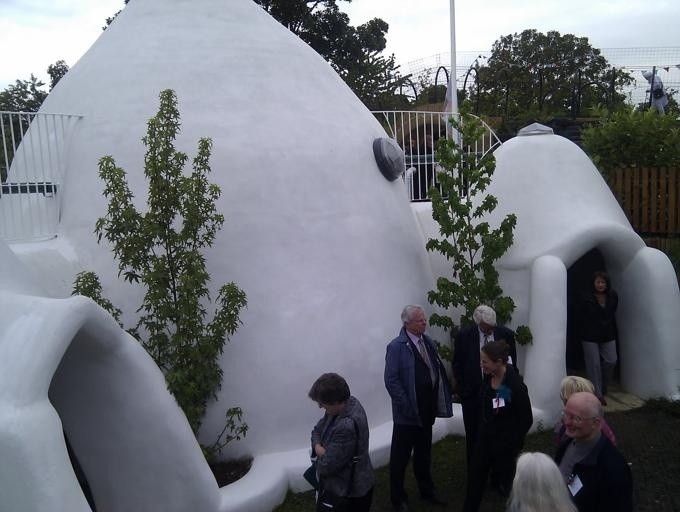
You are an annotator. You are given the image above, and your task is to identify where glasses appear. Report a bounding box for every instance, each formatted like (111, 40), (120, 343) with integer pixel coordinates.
(561, 410), (596, 424)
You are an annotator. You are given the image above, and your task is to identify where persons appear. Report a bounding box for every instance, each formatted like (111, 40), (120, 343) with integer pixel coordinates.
(403, 135), (472, 202)
(640, 69), (670, 119)
(501, 450), (580, 511)
(306, 372), (376, 511)
(383, 302), (456, 511)
(456, 338), (534, 512)
(550, 374), (619, 460)
(573, 269), (621, 407)
(450, 304), (534, 497)
(550, 393), (637, 511)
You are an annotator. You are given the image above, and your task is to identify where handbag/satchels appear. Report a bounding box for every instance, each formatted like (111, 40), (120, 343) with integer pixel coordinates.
(303, 462), (347, 509)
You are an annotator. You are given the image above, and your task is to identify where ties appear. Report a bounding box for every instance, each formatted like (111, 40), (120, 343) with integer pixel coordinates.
(418, 341), (435, 391)
